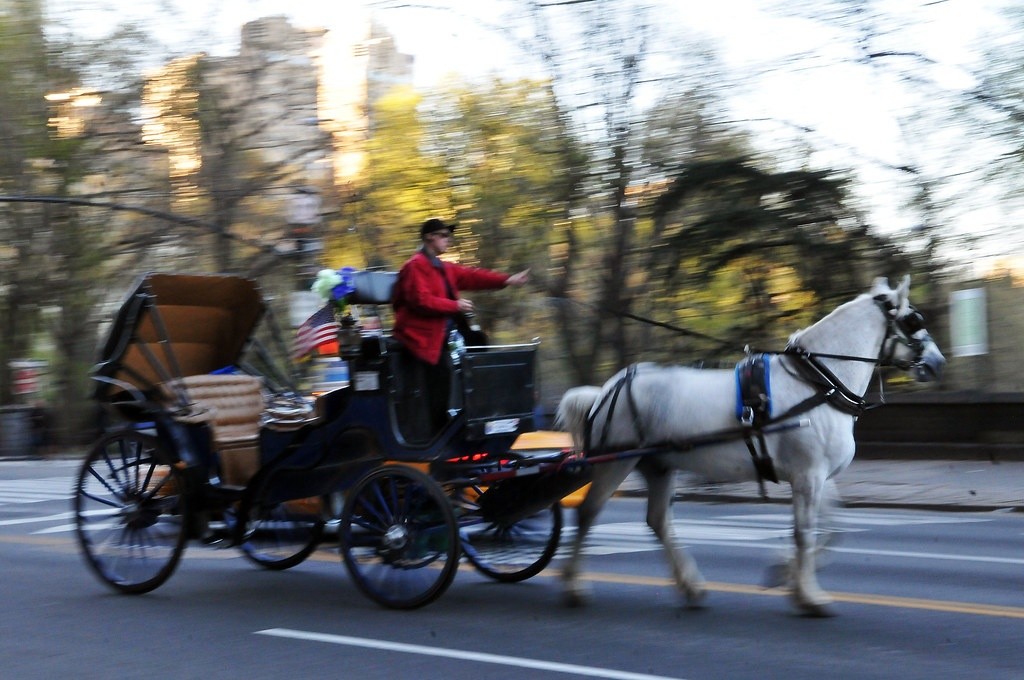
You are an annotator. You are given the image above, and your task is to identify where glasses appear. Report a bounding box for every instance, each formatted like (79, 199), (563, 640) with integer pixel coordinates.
(430, 232), (448, 237)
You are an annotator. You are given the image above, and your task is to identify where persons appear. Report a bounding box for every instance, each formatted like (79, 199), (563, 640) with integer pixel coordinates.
(392, 219), (532, 437)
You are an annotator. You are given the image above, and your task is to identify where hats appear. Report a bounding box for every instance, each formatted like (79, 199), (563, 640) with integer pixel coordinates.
(421, 219), (455, 234)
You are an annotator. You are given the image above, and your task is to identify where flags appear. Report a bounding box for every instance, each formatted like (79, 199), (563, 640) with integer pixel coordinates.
(355, 306), (384, 336)
(294, 305), (339, 358)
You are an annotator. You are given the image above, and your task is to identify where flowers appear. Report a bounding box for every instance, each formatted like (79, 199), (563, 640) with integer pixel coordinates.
(312, 269), (348, 312)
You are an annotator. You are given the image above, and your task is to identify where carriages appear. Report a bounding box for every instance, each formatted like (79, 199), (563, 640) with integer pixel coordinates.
(71, 217), (952, 620)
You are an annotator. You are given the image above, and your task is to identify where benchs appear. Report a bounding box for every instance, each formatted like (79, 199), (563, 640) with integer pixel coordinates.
(344, 330), (416, 400)
(165, 373), (348, 450)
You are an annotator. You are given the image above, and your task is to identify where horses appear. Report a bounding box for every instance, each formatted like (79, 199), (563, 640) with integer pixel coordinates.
(554, 274), (947, 619)
